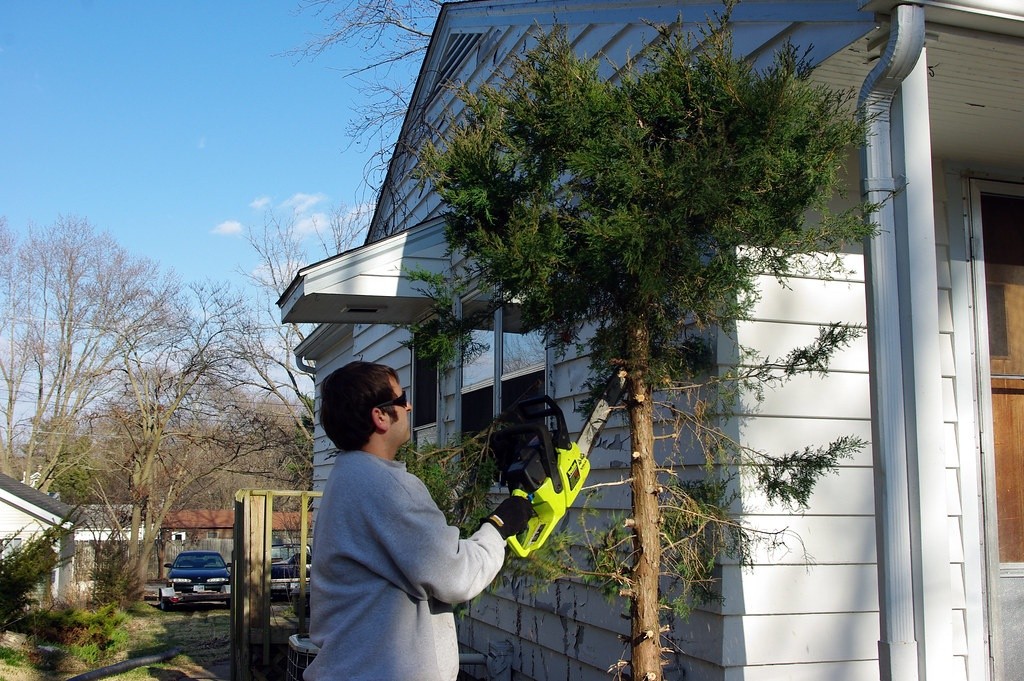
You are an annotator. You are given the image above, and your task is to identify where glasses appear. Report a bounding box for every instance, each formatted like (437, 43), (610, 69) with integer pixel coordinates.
(374, 390), (407, 408)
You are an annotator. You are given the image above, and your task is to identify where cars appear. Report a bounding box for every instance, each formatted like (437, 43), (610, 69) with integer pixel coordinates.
(163, 550), (232, 611)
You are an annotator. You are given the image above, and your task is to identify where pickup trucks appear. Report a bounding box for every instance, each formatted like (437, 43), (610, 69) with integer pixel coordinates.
(270, 544), (313, 598)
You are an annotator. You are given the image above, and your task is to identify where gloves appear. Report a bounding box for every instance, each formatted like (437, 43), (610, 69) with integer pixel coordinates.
(479, 496), (533, 539)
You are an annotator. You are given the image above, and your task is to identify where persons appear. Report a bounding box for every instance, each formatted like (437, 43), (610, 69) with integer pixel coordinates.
(303, 360), (534, 680)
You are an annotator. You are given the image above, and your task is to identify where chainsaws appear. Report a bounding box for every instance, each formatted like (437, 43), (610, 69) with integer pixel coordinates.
(487, 365), (631, 558)
